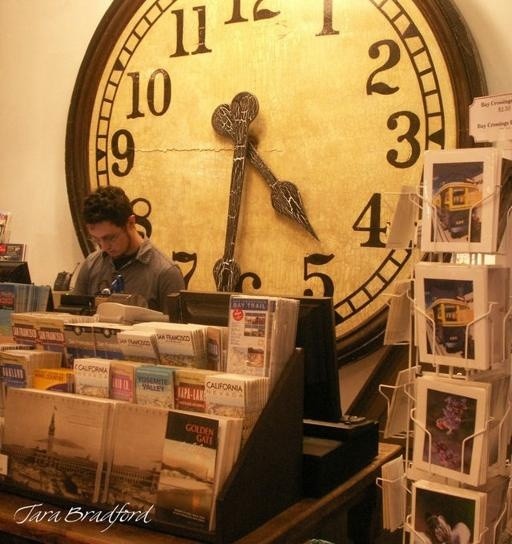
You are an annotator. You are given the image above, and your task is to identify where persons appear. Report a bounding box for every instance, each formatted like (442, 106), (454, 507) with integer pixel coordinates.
(70, 183), (186, 310)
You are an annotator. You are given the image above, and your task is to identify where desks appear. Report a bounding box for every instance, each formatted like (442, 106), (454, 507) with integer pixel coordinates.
(1, 442), (399, 543)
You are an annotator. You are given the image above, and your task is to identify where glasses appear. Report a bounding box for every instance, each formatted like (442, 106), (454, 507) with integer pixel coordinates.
(88, 225), (124, 243)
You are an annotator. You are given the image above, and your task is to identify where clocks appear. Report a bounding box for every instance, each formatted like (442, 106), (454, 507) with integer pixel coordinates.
(62, 0), (493, 368)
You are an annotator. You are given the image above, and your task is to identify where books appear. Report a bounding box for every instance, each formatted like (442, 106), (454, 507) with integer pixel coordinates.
(0, 281), (302, 535)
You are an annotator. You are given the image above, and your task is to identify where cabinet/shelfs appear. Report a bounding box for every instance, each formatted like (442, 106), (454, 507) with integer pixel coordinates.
(371, 92), (511, 544)
